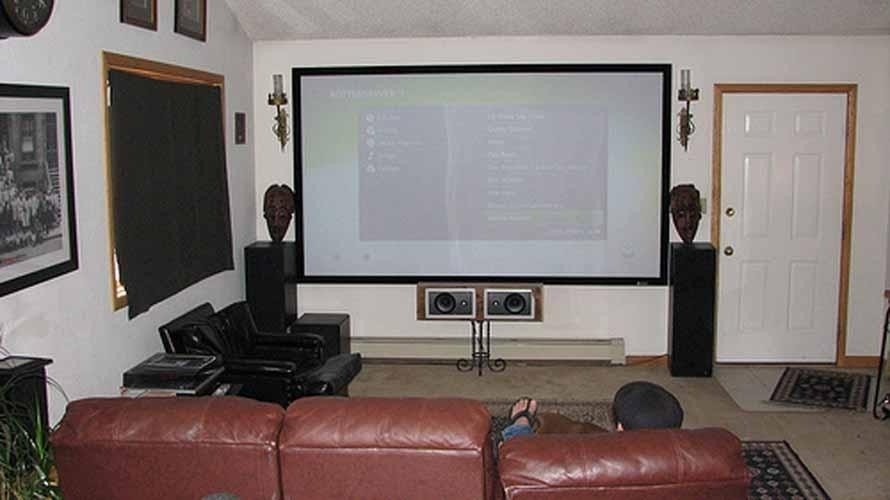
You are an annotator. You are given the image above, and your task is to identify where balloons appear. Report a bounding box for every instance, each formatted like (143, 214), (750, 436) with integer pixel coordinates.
(1, 1), (56, 39)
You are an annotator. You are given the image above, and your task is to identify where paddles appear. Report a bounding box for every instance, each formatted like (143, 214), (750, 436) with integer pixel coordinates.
(768, 365), (874, 415)
(739, 438), (836, 500)
(468, 398), (624, 435)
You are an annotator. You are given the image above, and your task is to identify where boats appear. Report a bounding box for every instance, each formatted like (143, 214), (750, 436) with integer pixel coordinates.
(667, 242), (717, 377)
(416, 282), (544, 323)
(244, 241), (298, 334)
(293, 312), (351, 365)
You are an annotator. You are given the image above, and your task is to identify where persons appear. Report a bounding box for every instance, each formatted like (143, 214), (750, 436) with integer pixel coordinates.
(499, 379), (686, 446)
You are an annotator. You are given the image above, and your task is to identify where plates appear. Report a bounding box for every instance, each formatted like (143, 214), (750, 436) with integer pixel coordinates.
(0, 353), (54, 477)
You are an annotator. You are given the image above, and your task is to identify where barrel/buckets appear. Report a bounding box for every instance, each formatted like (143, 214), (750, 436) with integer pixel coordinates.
(509, 397), (538, 427)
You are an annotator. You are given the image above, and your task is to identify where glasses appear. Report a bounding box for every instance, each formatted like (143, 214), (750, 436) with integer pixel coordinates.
(268, 73), (290, 150)
(676, 69), (699, 150)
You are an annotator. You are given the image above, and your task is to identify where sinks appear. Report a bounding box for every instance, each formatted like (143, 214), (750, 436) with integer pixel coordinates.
(154, 295), (364, 399)
(43, 392), (280, 500)
(282, 391), (492, 497)
(492, 418), (756, 499)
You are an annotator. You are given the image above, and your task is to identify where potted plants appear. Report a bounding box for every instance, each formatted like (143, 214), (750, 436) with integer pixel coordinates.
(117, 1), (159, 32)
(171, 0), (210, 45)
(0, 82), (80, 301)
